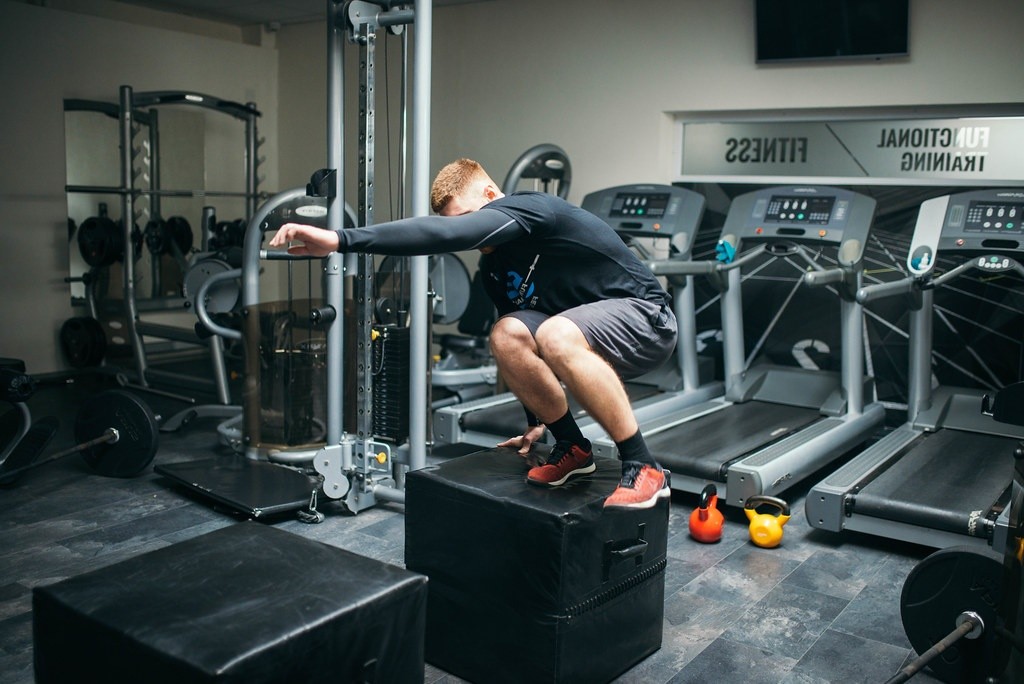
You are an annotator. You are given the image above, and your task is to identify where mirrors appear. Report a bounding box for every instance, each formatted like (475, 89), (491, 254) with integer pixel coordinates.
(61, 91), (207, 315)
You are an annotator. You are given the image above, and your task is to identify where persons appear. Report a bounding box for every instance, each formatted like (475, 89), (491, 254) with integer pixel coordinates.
(268, 159), (681, 511)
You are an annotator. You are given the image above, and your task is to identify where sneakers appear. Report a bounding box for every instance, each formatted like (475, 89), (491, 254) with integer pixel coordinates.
(603, 460), (672, 514)
(527, 437), (597, 488)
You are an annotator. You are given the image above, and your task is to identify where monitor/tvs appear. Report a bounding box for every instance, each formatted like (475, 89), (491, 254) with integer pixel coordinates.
(754, 0), (911, 65)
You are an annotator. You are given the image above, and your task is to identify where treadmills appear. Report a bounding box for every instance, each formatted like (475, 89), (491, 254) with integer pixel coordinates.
(588, 185), (887, 510)
(433, 184), (725, 449)
(805, 189), (1024, 567)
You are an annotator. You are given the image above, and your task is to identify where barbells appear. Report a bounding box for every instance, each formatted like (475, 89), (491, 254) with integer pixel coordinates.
(0, 389), (159, 480)
(880, 545), (1006, 684)
